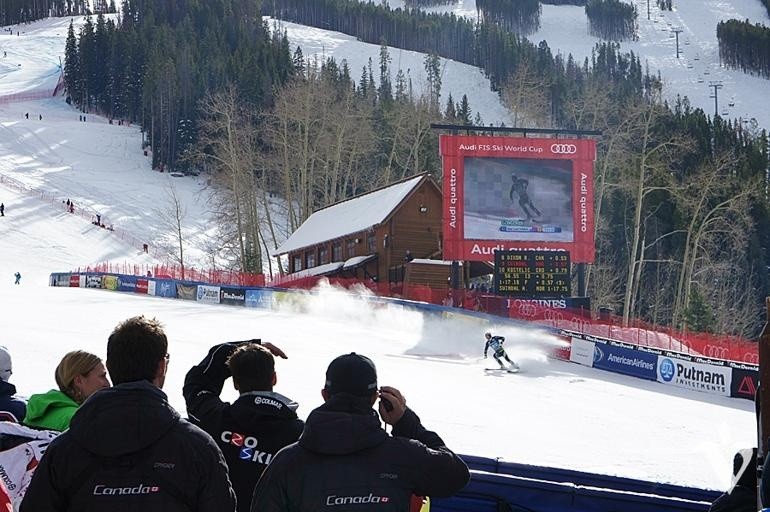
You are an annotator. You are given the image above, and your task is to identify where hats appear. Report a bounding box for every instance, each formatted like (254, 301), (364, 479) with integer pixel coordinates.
(325, 352), (384, 399)
(0, 344), (13, 380)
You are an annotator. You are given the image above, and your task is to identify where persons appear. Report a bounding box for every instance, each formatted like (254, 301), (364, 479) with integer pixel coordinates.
(80, 115), (82, 121)
(143, 244), (148, 254)
(26, 112), (28, 118)
(84, 116), (86, 122)
(510, 172), (543, 219)
(709, 387), (758, 512)
(0, 204), (4, 216)
(40, 114), (42, 120)
(64, 197), (116, 232)
(484, 332), (519, 370)
(14, 271), (20, 284)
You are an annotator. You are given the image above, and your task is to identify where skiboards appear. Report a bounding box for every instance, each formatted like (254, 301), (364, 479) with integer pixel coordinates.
(498, 218), (563, 232)
(484, 369), (528, 374)
